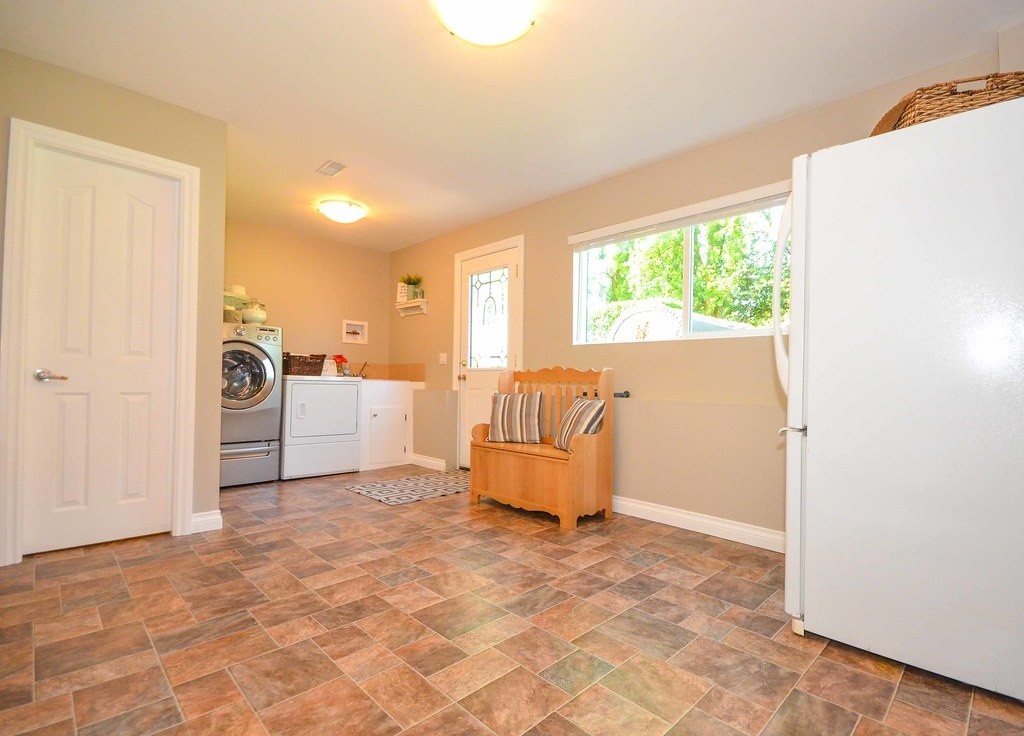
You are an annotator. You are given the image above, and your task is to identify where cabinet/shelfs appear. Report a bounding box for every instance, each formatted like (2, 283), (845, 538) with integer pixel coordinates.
(279, 375), (363, 480)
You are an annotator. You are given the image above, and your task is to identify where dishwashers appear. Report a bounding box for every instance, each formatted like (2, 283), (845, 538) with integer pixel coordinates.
(281, 374), (362, 480)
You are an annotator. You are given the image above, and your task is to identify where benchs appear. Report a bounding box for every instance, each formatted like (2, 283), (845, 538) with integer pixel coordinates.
(469, 365), (615, 530)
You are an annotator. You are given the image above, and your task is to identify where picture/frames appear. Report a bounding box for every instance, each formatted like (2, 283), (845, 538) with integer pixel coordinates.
(341, 319), (369, 344)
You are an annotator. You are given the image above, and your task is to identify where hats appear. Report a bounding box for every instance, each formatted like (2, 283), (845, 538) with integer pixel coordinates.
(224, 285), (250, 300)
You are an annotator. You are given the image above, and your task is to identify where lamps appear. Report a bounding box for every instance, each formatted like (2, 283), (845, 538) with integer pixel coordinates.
(428, 0), (542, 47)
(317, 196), (368, 223)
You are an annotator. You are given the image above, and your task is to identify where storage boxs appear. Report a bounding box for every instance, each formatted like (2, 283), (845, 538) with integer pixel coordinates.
(282, 351), (327, 376)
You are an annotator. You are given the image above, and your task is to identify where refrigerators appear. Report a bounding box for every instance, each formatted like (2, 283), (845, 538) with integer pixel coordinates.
(770, 96), (1024, 701)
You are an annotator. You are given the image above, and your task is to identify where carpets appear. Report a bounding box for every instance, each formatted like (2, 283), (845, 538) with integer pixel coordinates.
(344, 469), (471, 506)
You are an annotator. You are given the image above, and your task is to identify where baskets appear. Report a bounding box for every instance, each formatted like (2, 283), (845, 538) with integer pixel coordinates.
(892, 71), (1024, 130)
(282, 352), (327, 376)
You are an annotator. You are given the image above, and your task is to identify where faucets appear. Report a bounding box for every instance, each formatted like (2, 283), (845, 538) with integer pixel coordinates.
(358, 362), (370, 376)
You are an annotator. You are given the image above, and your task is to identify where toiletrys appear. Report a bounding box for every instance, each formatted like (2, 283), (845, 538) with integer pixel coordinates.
(342, 363), (352, 377)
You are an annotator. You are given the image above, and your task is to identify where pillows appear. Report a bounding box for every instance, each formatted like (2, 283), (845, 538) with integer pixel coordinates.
(484, 390), (542, 444)
(553, 395), (606, 454)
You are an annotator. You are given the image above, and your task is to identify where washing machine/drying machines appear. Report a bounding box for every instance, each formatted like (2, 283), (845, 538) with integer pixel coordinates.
(219, 322), (283, 490)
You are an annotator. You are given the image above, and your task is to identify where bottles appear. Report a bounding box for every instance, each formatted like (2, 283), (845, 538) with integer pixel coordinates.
(343, 363), (350, 377)
